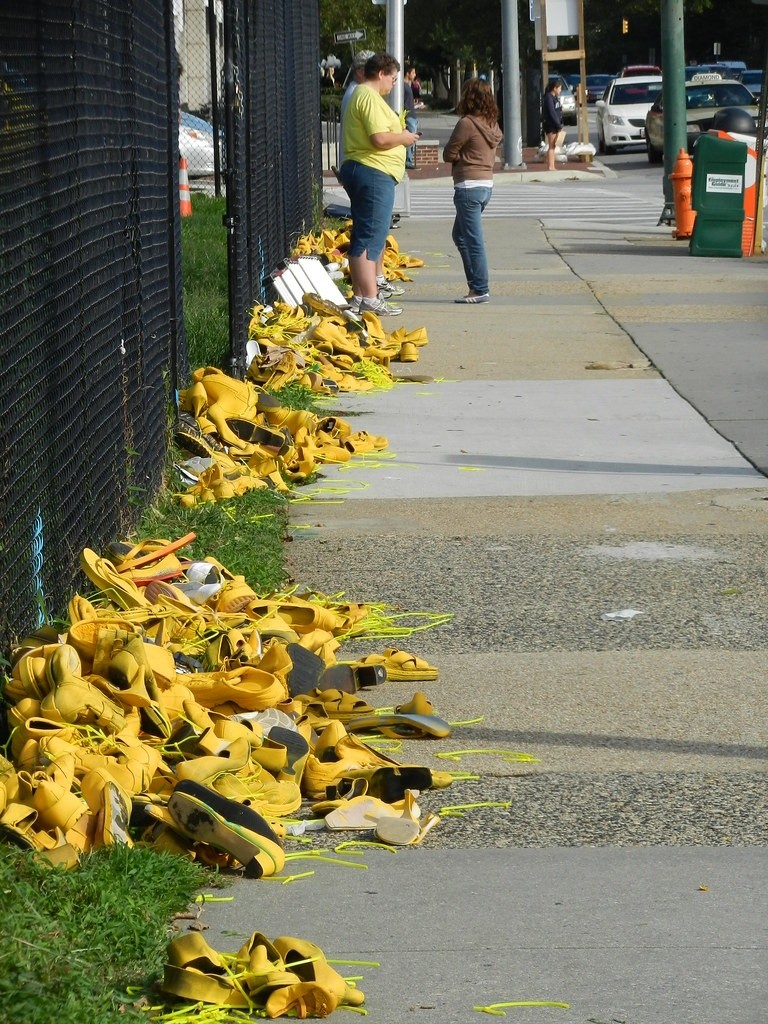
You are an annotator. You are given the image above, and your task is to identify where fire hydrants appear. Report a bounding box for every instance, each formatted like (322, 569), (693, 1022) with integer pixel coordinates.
(667, 147), (698, 240)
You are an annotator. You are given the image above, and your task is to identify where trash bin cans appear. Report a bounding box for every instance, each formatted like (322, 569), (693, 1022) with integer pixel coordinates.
(689, 134), (748, 258)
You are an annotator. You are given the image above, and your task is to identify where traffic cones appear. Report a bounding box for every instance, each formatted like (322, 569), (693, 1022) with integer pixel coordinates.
(178, 156), (192, 217)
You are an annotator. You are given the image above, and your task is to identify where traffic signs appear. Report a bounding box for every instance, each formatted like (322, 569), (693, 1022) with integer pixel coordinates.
(333, 28), (366, 45)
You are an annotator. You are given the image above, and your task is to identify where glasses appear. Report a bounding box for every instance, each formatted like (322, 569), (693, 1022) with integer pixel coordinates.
(389, 73), (398, 83)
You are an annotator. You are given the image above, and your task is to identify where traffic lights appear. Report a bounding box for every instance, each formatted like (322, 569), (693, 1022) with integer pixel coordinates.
(623, 16), (629, 34)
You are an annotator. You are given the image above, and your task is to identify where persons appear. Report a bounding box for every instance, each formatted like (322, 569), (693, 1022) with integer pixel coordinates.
(344, 53), (419, 316)
(543, 80), (564, 170)
(411, 77), (421, 103)
(403, 64), (422, 170)
(443, 79), (502, 303)
(339, 51), (405, 299)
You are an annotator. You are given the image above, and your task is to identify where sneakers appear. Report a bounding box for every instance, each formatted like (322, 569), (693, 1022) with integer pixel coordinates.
(377, 280), (405, 296)
(377, 285), (393, 298)
(350, 296), (362, 312)
(358, 293), (403, 316)
(454, 290), (489, 303)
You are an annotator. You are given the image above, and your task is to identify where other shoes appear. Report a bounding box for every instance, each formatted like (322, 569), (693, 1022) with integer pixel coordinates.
(0, 532), (453, 880)
(236, 930), (364, 1018)
(172, 366), (388, 507)
(290, 220), (424, 283)
(241, 302), (428, 394)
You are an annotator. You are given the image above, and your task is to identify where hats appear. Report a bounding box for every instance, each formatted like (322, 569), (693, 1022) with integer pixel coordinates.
(353, 50), (375, 69)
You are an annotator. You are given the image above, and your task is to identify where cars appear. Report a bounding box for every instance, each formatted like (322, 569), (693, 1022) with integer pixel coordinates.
(575, 73), (618, 104)
(541, 74), (578, 128)
(179, 111), (226, 180)
(595, 74), (663, 155)
(684, 61), (764, 105)
(617, 65), (662, 103)
(644, 72), (765, 163)
